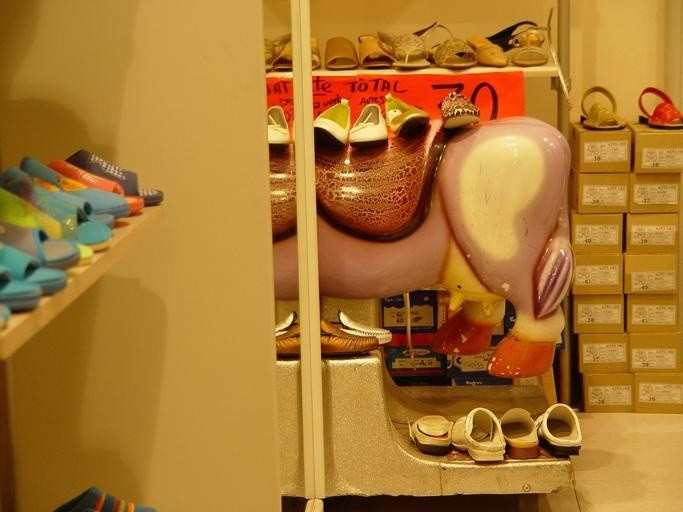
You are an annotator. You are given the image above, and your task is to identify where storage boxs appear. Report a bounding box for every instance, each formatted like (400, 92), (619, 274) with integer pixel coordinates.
(571, 122), (631, 173)
(579, 372), (633, 413)
(380, 291), (435, 333)
(446, 334), (512, 386)
(634, 371), (683, 414)
(576, 333), (628, 373)
(507, 304), (565, 350)
(384, 333), (448, 380)
(572, 253), (624, 295)
(624, 253), (683, 295)
(571, 214), (623, 253)
(570, 173), (630, 214)
(438, 287), (505, 337)
(626, 213), (683, 253)
(554, 365), (579, 407)
(573, 294), (626, 334)
(628, 121), (683, 173)
(630, 174), (683, 213)
(627, 294), (683, 333)
(628, 334), (683, 372)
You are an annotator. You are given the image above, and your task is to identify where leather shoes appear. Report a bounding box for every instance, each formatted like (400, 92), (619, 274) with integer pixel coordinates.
(350, 104), (388, 146)
(321, 319), (379, 355)
(276, 324), (300, 358)
(385, 93), (429, 135)
(267, 106), (290, 144)
(332, 311), (392, 345)
(314, 99), (350, 147)
(441, 92), (480, 129)
(275, 312), (297, 336)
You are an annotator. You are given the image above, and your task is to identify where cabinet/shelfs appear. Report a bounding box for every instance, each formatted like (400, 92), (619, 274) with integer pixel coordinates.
(269, 115), (575, 512)
(260, 0), (571, 407)
(1, 0), (324, 511)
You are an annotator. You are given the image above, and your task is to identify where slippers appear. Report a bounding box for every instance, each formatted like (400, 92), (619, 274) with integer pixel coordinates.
(537, 403), (581, 456)
(325, 37), (358, 69)
(1, 169), (115, 224)
(359, 35), (392, 67)
(0, 243), (66, 294)
(1, 269), (42, 311)
(1, 167), (111, 251)
(452, 407), (506, 461)
(0, 222), (78, 268)
(21, 157), (129, 218)
(1, 188), (94, 266)
(409, 415), (452, 455)
(501, 408), (539, 458)
(66, 150), (162, 206)
(54, 487), (154, 512)
(49, 159), (144, 215)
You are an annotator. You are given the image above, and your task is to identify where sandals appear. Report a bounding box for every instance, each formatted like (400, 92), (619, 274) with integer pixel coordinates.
(511, 25), (548, 67)
(430, 21), (478, 69)
(392, 33), (430, 68)
(581, 87), (625, 130)
(311, 38), (321, 70)
(272, 40), (293, 69)
(467, 35), (509, 67)
(639, 87), (683, 128)
